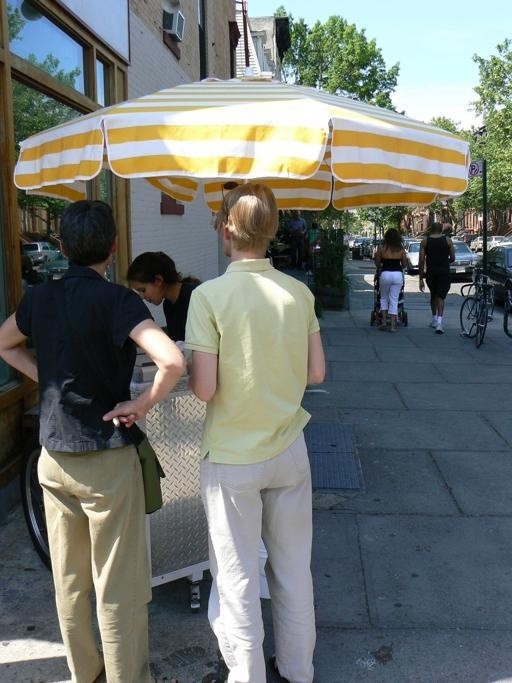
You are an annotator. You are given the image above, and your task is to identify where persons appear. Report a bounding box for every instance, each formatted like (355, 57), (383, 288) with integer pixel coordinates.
(124, 252), (203, 340)
(418, 221), (455, 334)
(374, 227), (408, 333)
(178, 184), (328, 682)
(1, 199), (189, 683)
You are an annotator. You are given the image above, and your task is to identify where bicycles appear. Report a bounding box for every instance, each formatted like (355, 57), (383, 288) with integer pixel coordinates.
(460, 261), (512, 348)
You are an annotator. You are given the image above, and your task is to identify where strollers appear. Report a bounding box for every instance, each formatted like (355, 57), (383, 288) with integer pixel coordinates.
(369, 268), (408, 327)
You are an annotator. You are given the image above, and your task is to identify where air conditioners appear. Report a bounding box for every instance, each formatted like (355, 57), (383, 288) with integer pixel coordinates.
(163, 9), (185, 42)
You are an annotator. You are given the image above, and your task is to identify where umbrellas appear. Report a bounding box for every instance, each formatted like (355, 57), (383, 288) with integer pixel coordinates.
(11, 64), (469, 222)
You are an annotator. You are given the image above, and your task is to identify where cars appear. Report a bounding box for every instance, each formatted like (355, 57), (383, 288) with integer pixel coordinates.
(347, 233), (511, 311)
(23, 240), (61, 261)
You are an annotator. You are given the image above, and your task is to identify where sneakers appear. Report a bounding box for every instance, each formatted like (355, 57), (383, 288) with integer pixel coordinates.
(432, 320), (444, 333)
(377, 322), (396, 332)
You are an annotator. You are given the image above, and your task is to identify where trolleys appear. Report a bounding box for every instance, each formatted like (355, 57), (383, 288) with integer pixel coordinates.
(20, 354), (214, 616)
